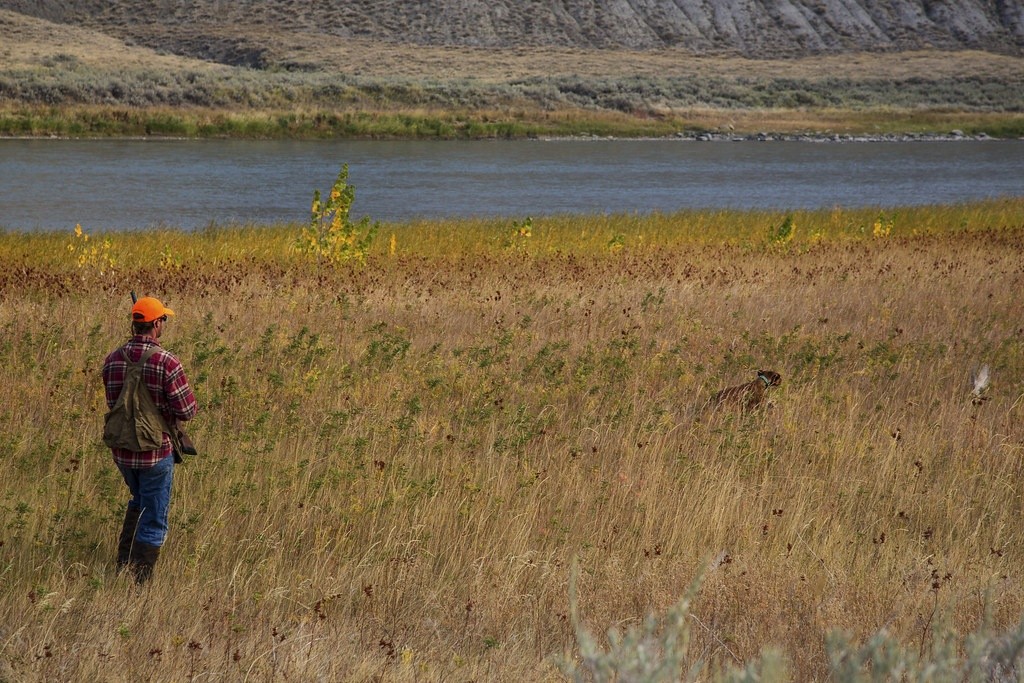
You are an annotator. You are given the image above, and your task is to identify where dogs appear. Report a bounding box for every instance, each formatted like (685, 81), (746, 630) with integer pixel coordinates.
(697, 368), (782, 413)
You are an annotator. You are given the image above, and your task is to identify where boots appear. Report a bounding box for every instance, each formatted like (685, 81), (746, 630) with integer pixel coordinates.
(116, 507), (142, 575)
(129, 539), (160, 586)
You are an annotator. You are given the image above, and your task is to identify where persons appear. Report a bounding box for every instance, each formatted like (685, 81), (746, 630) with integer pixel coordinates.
(101, 297), (197, 588)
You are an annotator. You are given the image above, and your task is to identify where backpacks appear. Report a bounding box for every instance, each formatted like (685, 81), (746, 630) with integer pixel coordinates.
(103, 346), (172, 452)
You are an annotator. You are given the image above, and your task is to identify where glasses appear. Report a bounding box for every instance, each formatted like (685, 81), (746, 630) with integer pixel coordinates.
(157, 314), (167, 321)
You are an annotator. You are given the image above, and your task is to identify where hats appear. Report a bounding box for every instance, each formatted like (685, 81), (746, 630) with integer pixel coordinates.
(131, 296), (174, 322)
